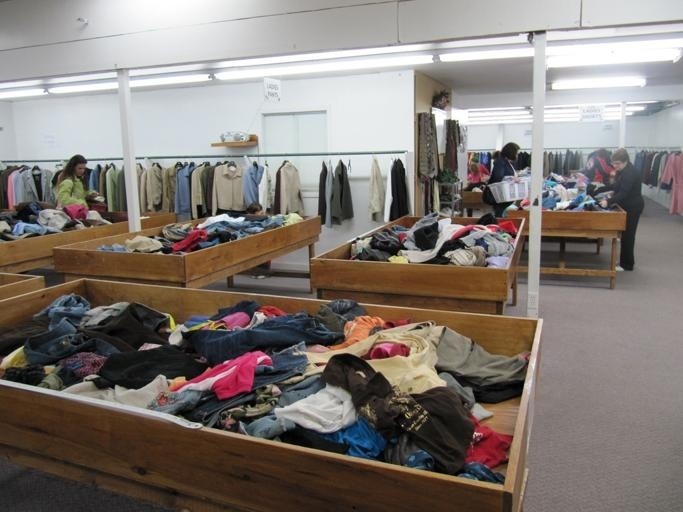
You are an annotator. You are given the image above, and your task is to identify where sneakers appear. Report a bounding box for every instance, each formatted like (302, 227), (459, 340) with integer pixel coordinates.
(615, 266), (626, 272)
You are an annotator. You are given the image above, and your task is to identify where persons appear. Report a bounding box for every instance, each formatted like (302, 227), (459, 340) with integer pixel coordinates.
(484, 142), (521, 217)
(56, 155), (99, 214)
(599, 148), (645, 272)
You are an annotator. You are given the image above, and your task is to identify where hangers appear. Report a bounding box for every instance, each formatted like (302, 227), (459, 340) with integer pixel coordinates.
(635, 144), (682, 153)
(467, 147), (621, 153)
(151, 159), (257, 167)
